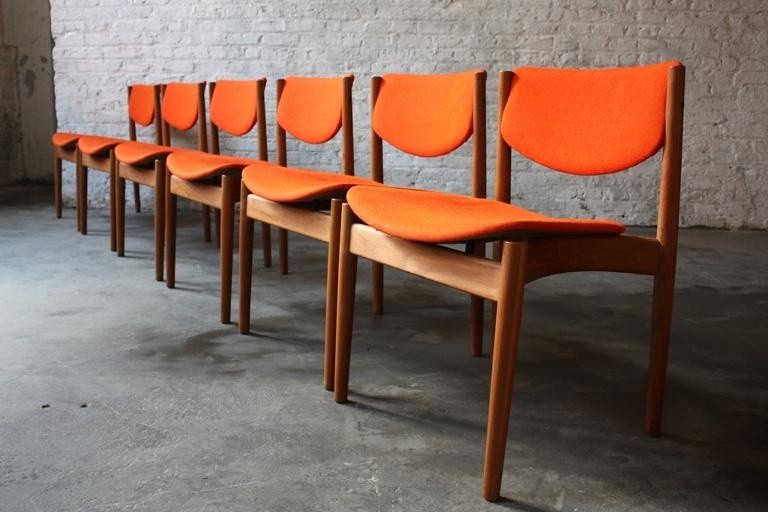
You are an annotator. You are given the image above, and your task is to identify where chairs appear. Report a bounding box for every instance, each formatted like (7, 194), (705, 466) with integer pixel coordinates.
(164, 74), (354, 324)
(114, 77), (273, 281)
(51, 84), (162, 233)
(240, 71), (489, 390)
(335, 61), (686, 499)
(77, 82), (206, 252)
